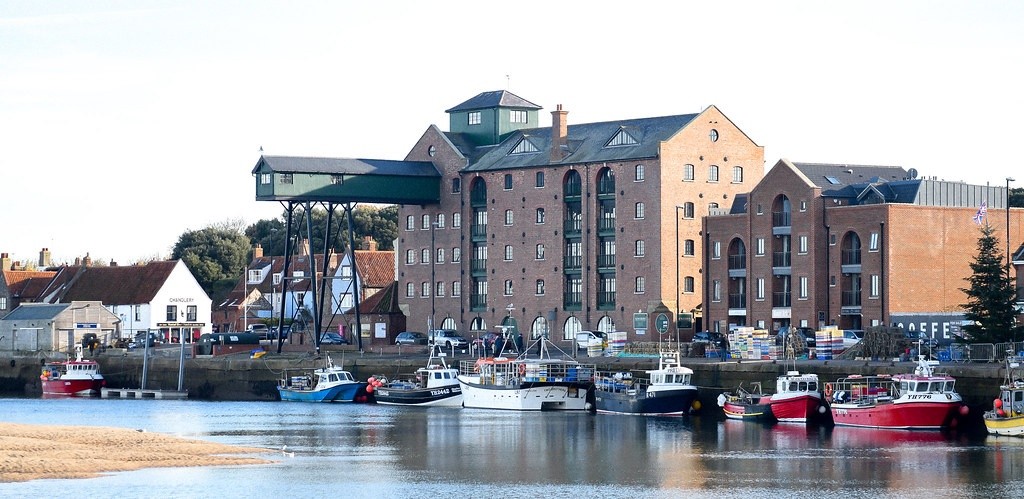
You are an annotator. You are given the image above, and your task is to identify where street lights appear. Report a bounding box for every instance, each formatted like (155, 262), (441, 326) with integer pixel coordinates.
(430, 221), (442, 329)
(269, 226), (278, 325)
(676, 204), (685, 327)
(1005, 177), (1016, 282)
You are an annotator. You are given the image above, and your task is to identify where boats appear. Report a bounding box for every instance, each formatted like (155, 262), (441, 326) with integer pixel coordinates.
(372, 338), (464, 407)
(455, 303), (596, 411)
(717, 380), (773, 421)
(983, 345), (1024, 437)
(38, 342), (107, 396)
(759, 330), (823, 422)
(595, 328), (699, 416)
(823, 332), (964, 430)
(276, 350), (369, 403)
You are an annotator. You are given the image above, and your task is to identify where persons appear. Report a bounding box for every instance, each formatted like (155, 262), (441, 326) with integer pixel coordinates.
(536, 332), (541, 356)
(88, 336), (94, 356)
(493, 335), (502, 357)
(516, 333), (523, 358)
(717, 333), (730, 362)
(482, 335), (490, 359)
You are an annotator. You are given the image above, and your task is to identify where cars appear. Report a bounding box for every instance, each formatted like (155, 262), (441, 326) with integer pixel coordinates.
(246, 323), (269, 339)
(270, 325), (292, 339)
(428, 329), (469, 349)
(396, 331), (428, 345)
(844, 328), (864, 348)
(313, 332), (351, 345)
(775, 326), (816, 346)
(472, 331), (512, 350)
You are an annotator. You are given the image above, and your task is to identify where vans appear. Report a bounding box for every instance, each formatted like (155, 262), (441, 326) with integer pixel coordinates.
(576, 329), (608, 350)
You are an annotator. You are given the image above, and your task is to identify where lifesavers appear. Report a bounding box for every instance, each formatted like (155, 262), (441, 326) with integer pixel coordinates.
(519, 364), (526, 375)
(825, 383), (832, 396)
